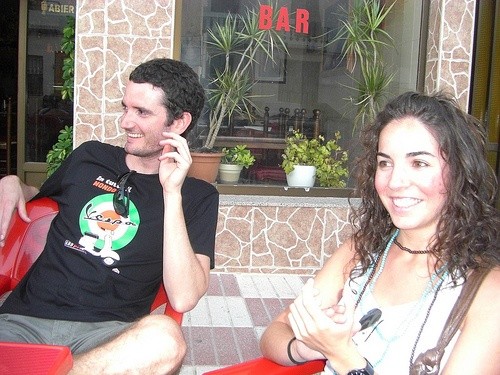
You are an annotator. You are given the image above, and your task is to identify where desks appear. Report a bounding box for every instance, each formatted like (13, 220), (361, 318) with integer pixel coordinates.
(197, 134), (285, 150)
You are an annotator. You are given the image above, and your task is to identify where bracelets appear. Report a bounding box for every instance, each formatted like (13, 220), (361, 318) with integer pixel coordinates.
(287, 337), (304, 364)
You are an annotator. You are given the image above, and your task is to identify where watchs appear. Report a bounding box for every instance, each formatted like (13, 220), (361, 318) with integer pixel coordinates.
(348, 357), (375, 375)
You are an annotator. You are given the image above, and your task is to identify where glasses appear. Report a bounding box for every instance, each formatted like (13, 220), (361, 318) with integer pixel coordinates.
(352, 308), (384, 346)
(113, 170), (137, 219)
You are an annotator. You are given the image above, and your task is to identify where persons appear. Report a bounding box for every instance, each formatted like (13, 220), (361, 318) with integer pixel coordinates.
(0, 58), (219, 375)
(31, 94), (66, 126)
(259, 92), (500, 375)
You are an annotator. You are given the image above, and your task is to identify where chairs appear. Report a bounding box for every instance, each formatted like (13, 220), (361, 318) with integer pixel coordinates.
(0, 196), (183, 329)
(0, 97), (17, 176)
(193, 106), (321, 184)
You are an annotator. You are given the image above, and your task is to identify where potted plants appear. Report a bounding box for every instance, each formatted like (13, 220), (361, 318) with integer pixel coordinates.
(216, 144), (256, 184)
(279, 127), (351, 190)
(184, 0), (302, 183)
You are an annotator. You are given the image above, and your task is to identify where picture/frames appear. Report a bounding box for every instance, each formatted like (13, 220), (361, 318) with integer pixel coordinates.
(251, 41), (288, 84)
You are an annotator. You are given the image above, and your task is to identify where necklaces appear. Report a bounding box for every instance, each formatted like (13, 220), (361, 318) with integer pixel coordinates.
(353, 228), (451, 375)
